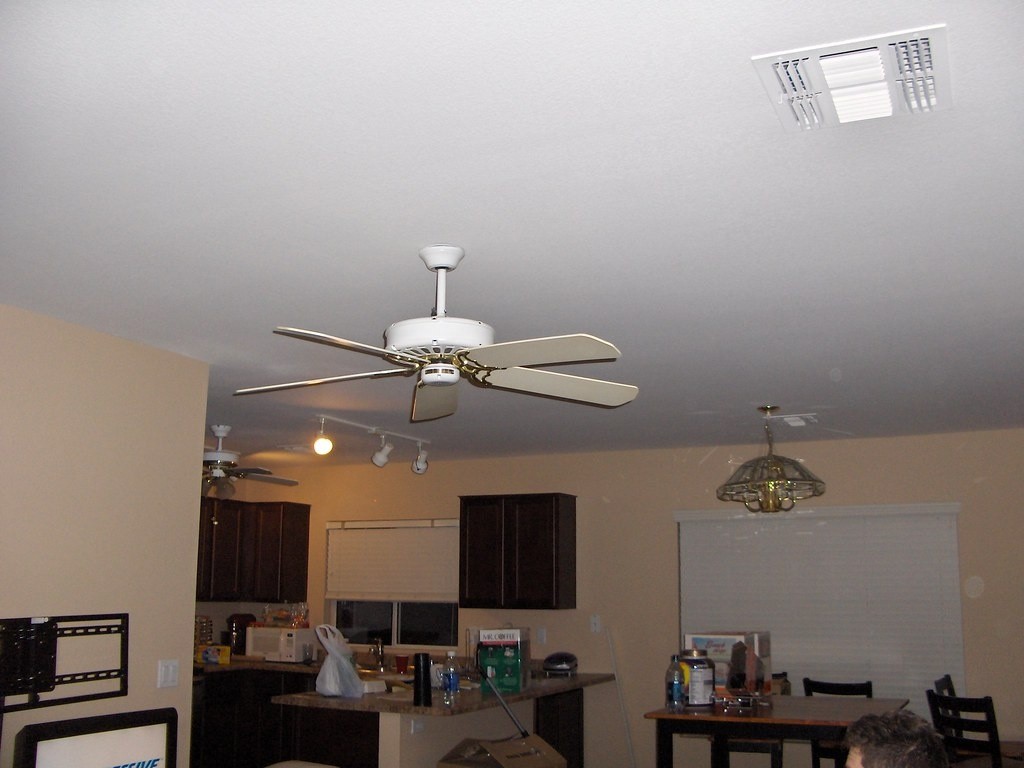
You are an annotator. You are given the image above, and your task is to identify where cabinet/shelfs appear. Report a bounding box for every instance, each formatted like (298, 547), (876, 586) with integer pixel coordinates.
(197, 496), (312, 604)
(190, 669), (380, 768)
(457, 492), (576, 611)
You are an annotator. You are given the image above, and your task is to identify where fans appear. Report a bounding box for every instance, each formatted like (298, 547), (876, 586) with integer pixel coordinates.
(203, 425), (299, 487)
(234, 244), (639, 421)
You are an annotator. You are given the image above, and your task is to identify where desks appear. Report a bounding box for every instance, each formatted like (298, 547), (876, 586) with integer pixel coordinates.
(643, 696), (909, 768)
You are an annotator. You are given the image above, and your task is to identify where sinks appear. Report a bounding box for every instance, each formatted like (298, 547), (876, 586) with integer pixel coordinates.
(357, 668), (383, 673)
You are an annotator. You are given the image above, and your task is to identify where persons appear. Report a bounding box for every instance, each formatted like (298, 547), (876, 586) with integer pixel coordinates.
(840, 709), (950, 768)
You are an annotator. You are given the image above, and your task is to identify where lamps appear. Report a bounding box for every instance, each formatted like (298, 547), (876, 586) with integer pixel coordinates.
(715, 405), (825, 513)
(410, 441), (428, 475)
(201, 476), (234, 525)
(314, 418), (333, 456)
(371, 435), (394, 468)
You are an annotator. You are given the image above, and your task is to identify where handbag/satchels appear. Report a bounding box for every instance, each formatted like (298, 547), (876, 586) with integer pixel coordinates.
(315, 625), (365, 699)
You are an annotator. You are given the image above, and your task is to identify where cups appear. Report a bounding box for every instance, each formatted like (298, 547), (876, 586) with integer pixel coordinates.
(394, 655), (410, 674)
(303, 642), (313, 664)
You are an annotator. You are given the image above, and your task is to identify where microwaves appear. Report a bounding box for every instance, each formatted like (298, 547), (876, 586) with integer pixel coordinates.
(245, 626), (321, 663)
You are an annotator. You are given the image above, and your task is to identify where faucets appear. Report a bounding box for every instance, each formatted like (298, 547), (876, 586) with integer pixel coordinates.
(368, 637), (384, 667)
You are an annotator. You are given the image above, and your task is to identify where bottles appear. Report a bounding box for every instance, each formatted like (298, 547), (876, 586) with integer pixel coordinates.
(680, 648), (715, 707)
(443, 652), (459, 705)
(664, 655), (685, 715)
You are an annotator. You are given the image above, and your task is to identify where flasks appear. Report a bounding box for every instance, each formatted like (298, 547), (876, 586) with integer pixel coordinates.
(413, 653), (433, 707)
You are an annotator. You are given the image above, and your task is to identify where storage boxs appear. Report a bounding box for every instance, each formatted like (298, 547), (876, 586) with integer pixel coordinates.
(771, 672), (792, 696)
(684, 629), (772, 698)
(476, 640), (533, 696)
(479, 626), (530, 641)
(436, 732), (567, 768)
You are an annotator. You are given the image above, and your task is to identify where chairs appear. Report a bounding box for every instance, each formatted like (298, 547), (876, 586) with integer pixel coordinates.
(803, 678), (872, 768)
(926, 673), (1024, 768)
(710, 672), (787, 768)
